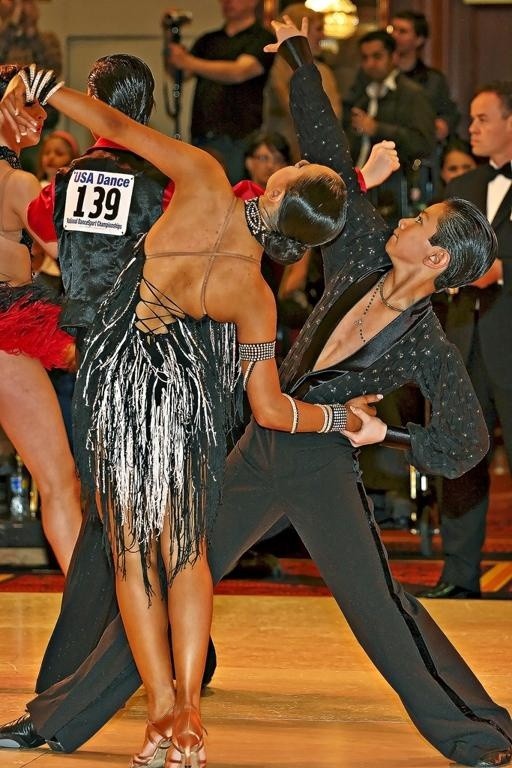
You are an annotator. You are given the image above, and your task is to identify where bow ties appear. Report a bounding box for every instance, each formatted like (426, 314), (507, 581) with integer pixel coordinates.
(485, 161), (512, 183)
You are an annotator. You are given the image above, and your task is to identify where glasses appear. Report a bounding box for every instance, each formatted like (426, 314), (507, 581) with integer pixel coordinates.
(251, 152), (284, 165)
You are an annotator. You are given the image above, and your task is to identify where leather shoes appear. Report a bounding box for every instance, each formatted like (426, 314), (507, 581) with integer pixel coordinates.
(413, 577), (481, 599)
(473, 749), (511, 768)
(0, 707), (66, 753)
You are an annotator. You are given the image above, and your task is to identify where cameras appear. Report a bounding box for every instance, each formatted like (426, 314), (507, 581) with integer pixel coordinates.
(162, 9), (193, 26)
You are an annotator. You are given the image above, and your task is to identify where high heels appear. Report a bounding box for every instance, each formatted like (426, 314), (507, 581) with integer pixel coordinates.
(162, 702), (209, 768)
(131, 705), (180, 768)
